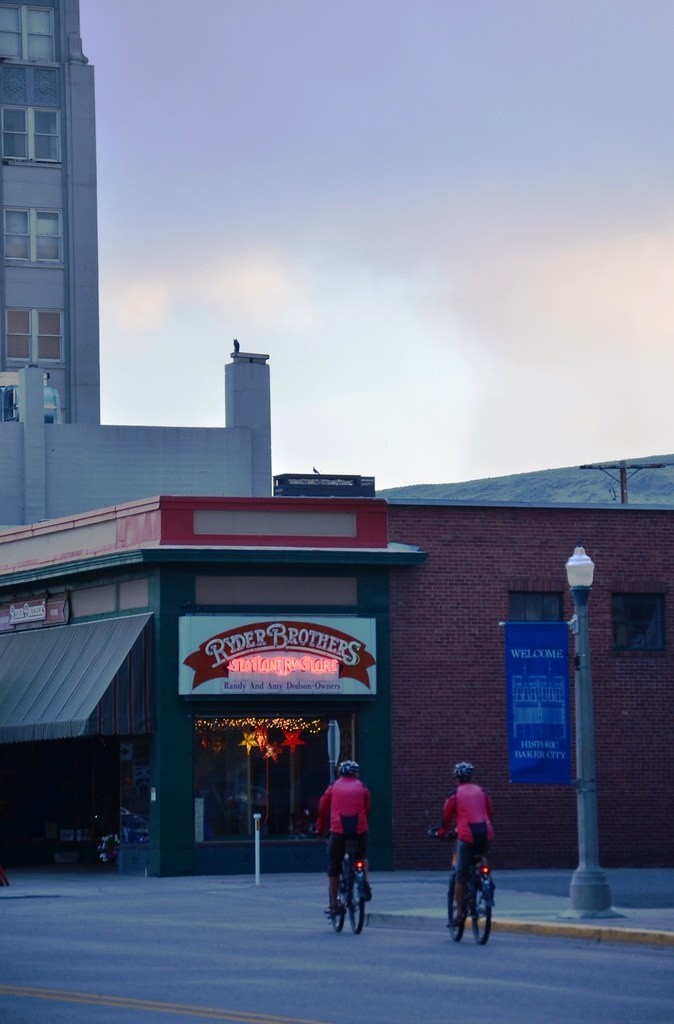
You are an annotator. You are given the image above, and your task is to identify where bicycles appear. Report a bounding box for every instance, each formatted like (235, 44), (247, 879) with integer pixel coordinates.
(304, 809), (369, 934)
(427, 829), (497, 947)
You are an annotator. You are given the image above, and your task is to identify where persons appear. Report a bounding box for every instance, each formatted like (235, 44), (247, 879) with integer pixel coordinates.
(429, 761), (495, 927)
(315, 761), (371, 914)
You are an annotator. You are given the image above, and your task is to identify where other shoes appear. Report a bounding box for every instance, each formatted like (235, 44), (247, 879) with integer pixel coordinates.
(364, 881), (372, 902)
(446, 915), (464, 927)
(324, 905), (338, 915)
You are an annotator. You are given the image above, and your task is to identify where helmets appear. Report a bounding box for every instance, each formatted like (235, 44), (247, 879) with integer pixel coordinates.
(453, 762), (475, 779)
(338, 760), (359, 777)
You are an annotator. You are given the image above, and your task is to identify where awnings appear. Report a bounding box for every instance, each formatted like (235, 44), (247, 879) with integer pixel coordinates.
(0, 612), (155, 743)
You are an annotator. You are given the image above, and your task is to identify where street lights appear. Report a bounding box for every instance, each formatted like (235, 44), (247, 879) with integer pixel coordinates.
(564, 545), (624, 920)
